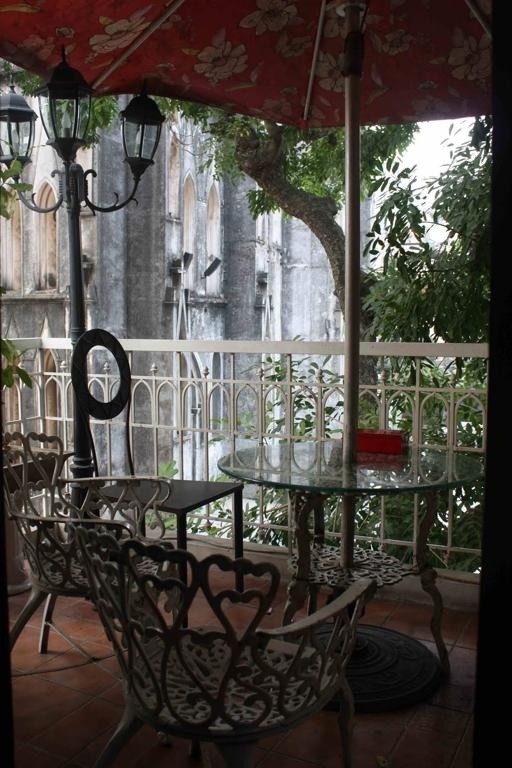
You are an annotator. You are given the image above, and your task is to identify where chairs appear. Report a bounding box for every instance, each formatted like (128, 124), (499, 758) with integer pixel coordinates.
(3, 433), (199, 746)
(64, 522), (378, 767)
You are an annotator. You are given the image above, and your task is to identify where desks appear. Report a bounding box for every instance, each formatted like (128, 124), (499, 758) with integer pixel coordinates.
(217, 439), (484, 716)
(96, 479), (244, 630)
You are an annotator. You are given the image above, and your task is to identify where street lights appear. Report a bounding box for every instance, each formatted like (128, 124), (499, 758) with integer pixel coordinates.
(0, 46), (166, 526)
